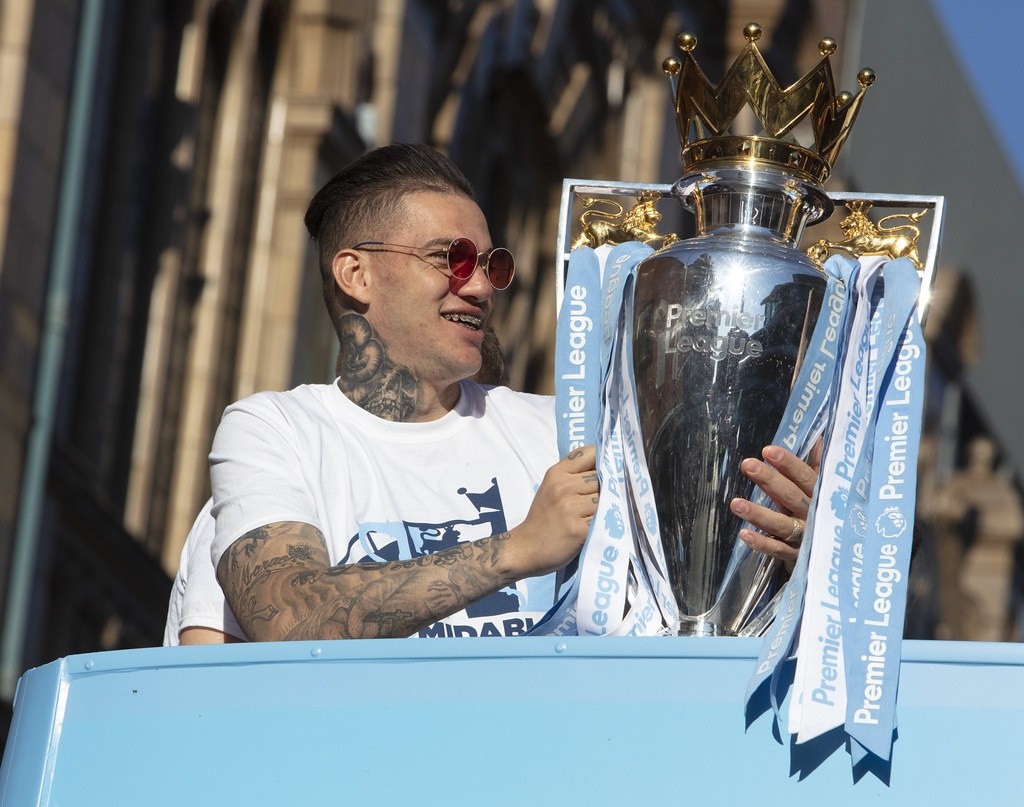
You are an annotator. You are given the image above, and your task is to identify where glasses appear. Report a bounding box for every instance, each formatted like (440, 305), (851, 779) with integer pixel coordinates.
(332, 238), (516, 296)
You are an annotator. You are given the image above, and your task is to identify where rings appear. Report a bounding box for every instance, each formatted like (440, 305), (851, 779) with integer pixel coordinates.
(785, 516), (803, 544)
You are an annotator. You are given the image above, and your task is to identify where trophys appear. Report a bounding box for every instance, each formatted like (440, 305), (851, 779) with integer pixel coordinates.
(555, 19), (944, 638)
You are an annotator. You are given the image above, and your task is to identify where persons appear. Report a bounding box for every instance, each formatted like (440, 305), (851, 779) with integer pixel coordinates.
(208, 141), (600, 638)
(162, 318), (511, 645)
